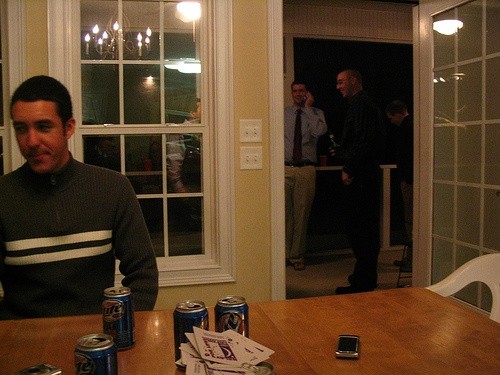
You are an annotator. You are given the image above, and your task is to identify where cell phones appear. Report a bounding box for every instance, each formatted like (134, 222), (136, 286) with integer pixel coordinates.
(335, 335), (359, 358)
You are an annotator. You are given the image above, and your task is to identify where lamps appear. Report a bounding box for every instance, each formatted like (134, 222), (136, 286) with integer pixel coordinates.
(85, 4), (152, 61)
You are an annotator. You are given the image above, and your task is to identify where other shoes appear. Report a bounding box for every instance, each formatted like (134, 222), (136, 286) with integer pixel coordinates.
(348, 274), (354, 284)
(337, 285), (374, 295)
(394, 259), (413, 273)
(293, 262), (305, 270)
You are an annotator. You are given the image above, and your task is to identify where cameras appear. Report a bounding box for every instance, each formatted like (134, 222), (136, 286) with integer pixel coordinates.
(15, 365), (64, 375)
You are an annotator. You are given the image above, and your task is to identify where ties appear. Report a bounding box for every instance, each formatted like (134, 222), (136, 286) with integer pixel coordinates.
(291, 109), (303, 163)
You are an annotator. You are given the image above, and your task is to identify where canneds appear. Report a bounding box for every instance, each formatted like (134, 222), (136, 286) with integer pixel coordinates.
(173, 300), (210, 372)
(214, 295), (249, 339)
(74, 333), (118, 375)
(102, 287), (136, 350)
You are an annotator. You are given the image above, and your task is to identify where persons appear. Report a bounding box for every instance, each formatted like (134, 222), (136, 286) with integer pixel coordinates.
(329, 70), (384, 294)
(284, 81), (326, 268)
(85, 136), (120, 171)
(0, 75), (160, 320)
(167, 98), (203, 234)
(387, 99), (414, 273)
(141, 141), (164, 183)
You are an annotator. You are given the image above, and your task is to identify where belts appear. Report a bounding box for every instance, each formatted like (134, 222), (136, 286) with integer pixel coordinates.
(284, 161), (312, 167)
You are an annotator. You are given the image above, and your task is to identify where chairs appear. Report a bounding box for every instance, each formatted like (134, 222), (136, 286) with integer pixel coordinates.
(424, 252), (500, 323)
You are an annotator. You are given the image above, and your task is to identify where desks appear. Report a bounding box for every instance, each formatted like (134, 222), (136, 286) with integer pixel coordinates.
(0, 287), (500, 375)
